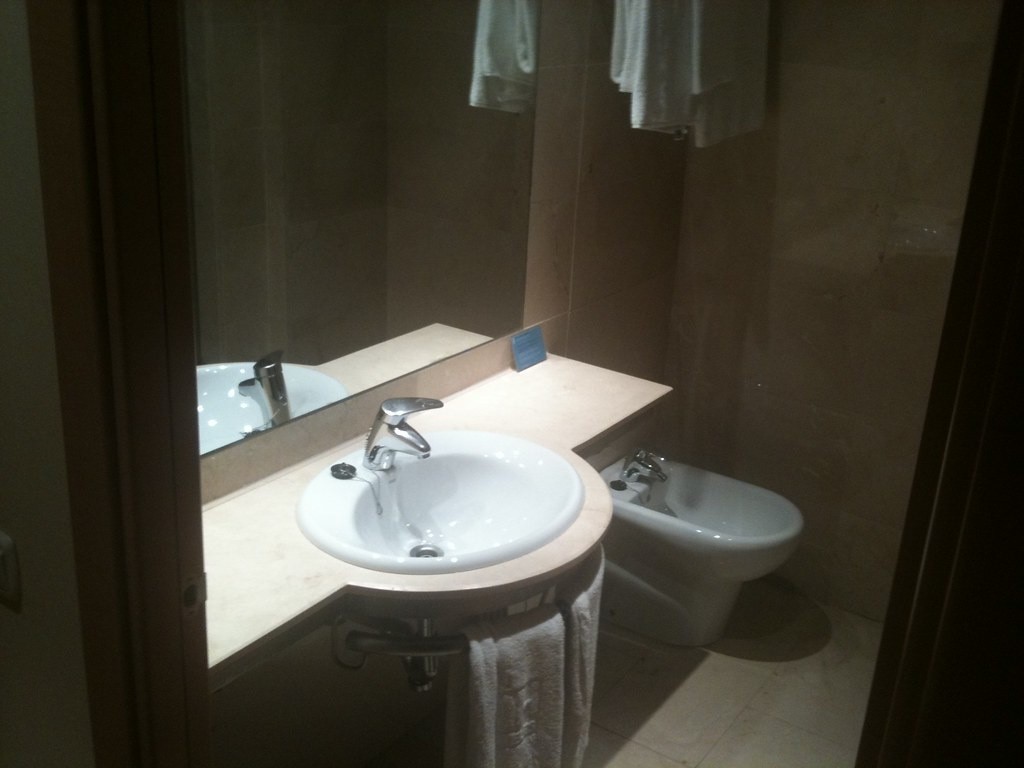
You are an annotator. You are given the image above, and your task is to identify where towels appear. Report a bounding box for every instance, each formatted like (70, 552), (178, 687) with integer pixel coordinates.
(445, 542), (606, 768)
(609, 0), (771, 148)
(469, 1), (540, 114)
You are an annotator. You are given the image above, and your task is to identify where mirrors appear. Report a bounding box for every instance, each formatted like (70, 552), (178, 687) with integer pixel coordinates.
(186, 0), (541, 456)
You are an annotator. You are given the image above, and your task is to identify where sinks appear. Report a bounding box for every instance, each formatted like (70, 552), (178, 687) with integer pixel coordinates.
(299, 430), (587, 574)
(197, 362), (349, 454)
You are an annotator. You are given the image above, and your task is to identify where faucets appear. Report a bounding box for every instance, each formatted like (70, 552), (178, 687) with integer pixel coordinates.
(365, 397), (445, 472)
(621, 446), (668, 485)
(237, 359), (292, 429)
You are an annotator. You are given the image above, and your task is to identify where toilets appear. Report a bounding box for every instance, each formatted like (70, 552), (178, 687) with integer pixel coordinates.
(598, 449), (805, 648)
